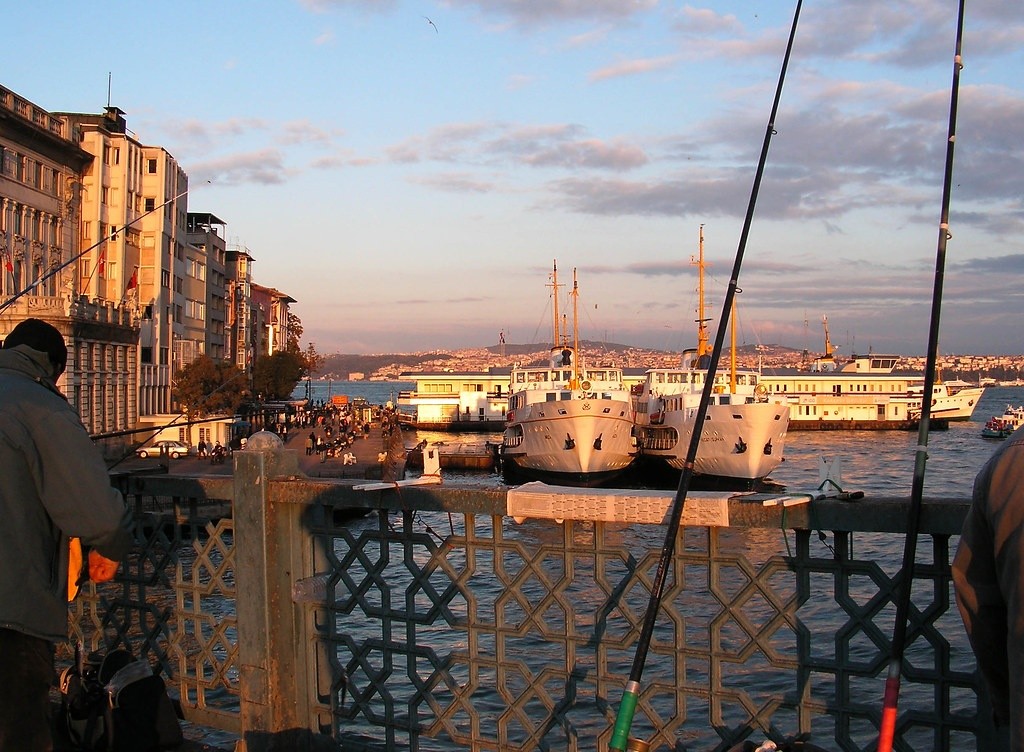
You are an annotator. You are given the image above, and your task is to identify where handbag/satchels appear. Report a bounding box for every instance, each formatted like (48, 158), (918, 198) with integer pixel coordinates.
(57, 647), (184, 752)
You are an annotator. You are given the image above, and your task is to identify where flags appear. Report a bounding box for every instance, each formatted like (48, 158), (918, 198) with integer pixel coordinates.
(126, 271), (137, 289)
(98, 253), (105, 274)
(5, 251), (13, 272)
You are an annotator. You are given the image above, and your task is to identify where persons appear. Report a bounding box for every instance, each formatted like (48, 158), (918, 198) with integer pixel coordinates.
(310, 398), (337, 427)
(295, 410), (316, 429)
(380, 414), (393, 438)
(952, 421), (1023, 751)
(338, 406), (371, 433)
(198, 438), (207, 460)
(269, 419), (288, 443)
(332, 431), (355, 447)
(229, 435), (240, 458)
(214, 440), (223, 464)
(0, 317), (138, 751)
(305, 431), (326, 463)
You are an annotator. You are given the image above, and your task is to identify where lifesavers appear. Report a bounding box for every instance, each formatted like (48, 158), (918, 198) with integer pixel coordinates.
(581, 380), (592, 391)
(834, 411), (838, 415)
(881, 422), (889, 429)
(900, 423), (908, 430)
(820, 422), (843, 430)
(755, 383), (768, 396)
(855, 424), (861, 430)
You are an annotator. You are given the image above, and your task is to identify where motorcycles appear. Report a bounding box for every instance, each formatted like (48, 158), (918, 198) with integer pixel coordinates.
(211, 447), (227, 464)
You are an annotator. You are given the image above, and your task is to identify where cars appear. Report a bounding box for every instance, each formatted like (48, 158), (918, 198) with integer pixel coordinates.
(135, 440), (190, 459)
(396, 422), (415, 431)
(354, 398), (366, 412)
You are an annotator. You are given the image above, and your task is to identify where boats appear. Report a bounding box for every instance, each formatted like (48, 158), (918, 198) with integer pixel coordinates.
(500, 259), (642, 488)
(632, 227), (790, 491)
(942, 380), (974, 389)
(980, 405), (1024, 439)
(1000, 380), (1024, 388)
(980, 378), (997, 387)
(395, 319), (986, 430)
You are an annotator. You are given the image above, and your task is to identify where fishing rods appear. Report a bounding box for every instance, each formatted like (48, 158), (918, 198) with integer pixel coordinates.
(1, 176), (212, 321)
(90, 409), (343, 439)
(109, 368), (246, 469)
(610, 0), (809, 752)
(880, 0), (967, 752)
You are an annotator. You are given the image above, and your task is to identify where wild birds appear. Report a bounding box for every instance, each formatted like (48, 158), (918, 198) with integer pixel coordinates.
(423, 16), (438, 34)
